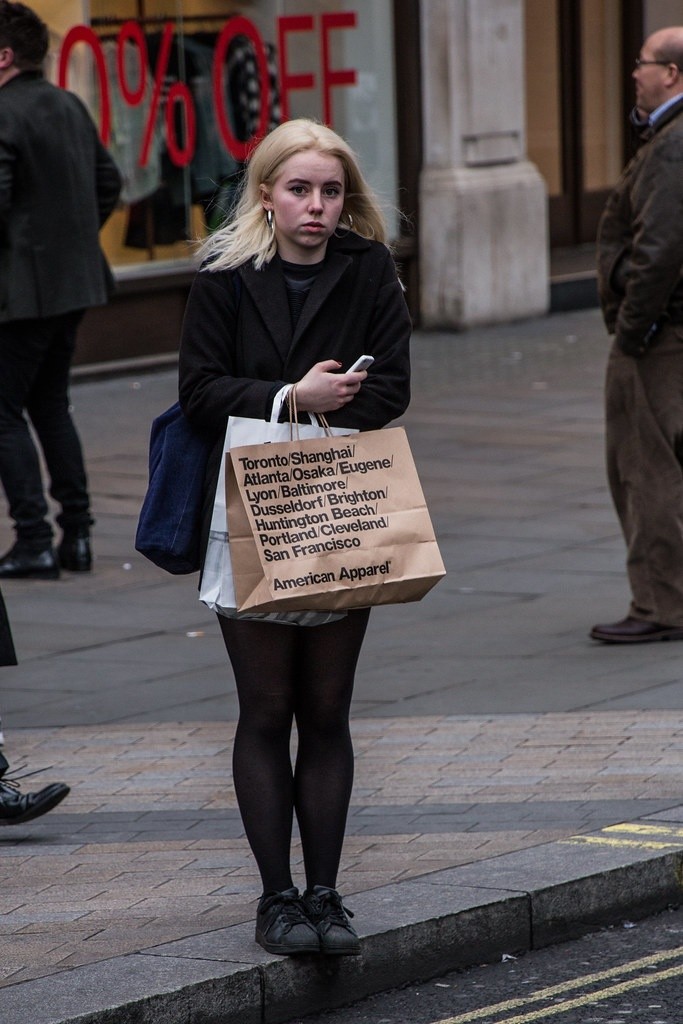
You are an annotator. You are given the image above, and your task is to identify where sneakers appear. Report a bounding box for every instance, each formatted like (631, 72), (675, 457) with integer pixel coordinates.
(255, 886), (321, 955)
(301, 885), (362, 955)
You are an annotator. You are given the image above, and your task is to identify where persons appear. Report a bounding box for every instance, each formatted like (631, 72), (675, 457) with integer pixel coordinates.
(0, 1), (123, 577)
(0, 740), (70, 826)
(589, 27), (682, 643)
(177, 121), (413, 957)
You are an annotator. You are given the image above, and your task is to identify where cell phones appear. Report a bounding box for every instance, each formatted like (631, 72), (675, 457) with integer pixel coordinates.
(345, 354), (375, 375)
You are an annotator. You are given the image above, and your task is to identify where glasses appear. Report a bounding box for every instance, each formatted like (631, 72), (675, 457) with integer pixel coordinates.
(633, 57), (670, 68)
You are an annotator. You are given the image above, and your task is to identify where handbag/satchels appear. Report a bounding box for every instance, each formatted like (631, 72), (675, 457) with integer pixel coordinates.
(199, 383), (446, 626)
(134, 401), (217, 575)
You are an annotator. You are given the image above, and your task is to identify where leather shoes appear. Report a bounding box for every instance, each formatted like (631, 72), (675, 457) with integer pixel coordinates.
(589, 615), (683, 644)
(0, 765), (70, 825)
(56, 535), (92, 573)
(0, 543), (60, 580)
